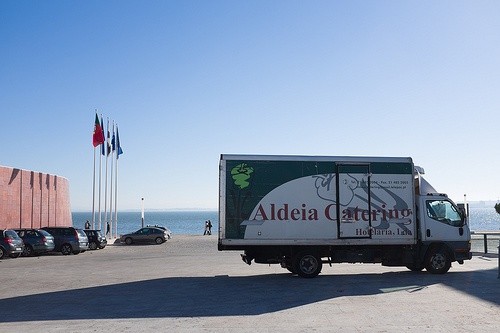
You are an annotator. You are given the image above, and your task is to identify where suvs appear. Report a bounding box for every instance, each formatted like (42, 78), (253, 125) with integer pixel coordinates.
(38, 226), (91, 255)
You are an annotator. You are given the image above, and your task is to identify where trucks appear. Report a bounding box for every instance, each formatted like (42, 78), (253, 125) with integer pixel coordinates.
(218, 153), (473, 279)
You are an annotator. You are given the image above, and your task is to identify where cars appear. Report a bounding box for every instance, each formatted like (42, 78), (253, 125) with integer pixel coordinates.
(12, 227), (56, 257)
(120, 223), (172, 245)
(0, 229), (26, 260)
(83, 229), (107, 250)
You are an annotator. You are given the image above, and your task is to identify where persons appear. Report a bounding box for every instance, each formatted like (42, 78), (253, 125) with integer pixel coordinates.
(106, 222), (110, 234)
(85, 220), (91, 229)
(204, 220), (211, 235)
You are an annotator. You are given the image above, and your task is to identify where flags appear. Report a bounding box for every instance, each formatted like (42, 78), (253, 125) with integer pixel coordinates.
(107, 124), (111, 156)
(101, 119), (105, 155)
(93, 114), (105, 146)
(111, 131), (115, 150)
(117, 129), (123, 159)
